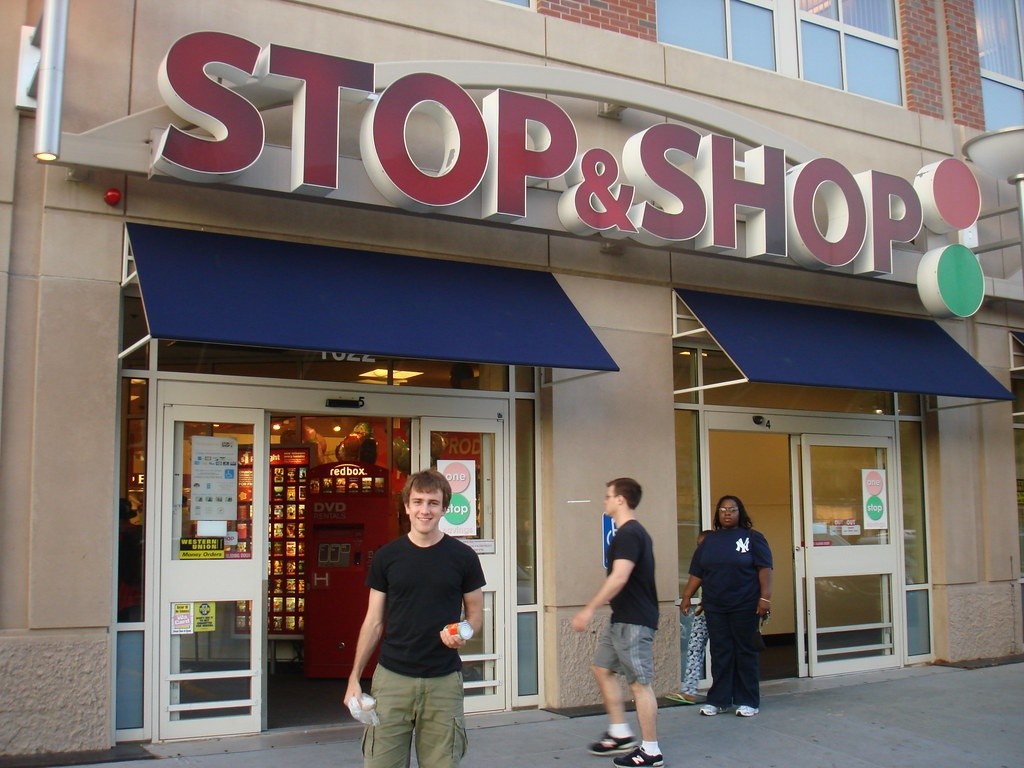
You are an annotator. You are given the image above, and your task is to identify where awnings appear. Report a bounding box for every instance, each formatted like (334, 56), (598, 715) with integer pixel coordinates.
(1009, 331), (1024, 371)
(671, 287), (1017, 401)
(125, 221), (620, 371)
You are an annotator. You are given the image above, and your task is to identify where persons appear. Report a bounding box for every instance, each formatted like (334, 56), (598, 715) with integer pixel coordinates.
(570, 477), (664, 768)
(664, 495), (773, 717)
(118, 496), (195, 587)
(344, 469), (486, 768)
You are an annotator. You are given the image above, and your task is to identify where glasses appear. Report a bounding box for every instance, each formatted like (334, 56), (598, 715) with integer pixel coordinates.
(718, 507), (739, 513)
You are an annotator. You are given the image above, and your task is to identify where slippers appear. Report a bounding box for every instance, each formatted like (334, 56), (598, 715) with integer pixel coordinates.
(665, 693), (696, 705)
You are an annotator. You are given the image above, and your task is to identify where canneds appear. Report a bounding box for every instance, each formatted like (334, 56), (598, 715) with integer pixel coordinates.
(443, 622), (473, 640)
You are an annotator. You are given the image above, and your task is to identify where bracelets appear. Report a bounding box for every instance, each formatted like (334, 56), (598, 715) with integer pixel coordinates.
(760, 597), (771, 604)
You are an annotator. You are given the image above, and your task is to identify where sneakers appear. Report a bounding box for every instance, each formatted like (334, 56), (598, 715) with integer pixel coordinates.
(700, 704), (728, 716)
(613, 746), (664, 768)
(735, 705), (759, 717)
(586, 732), (639, 756)
